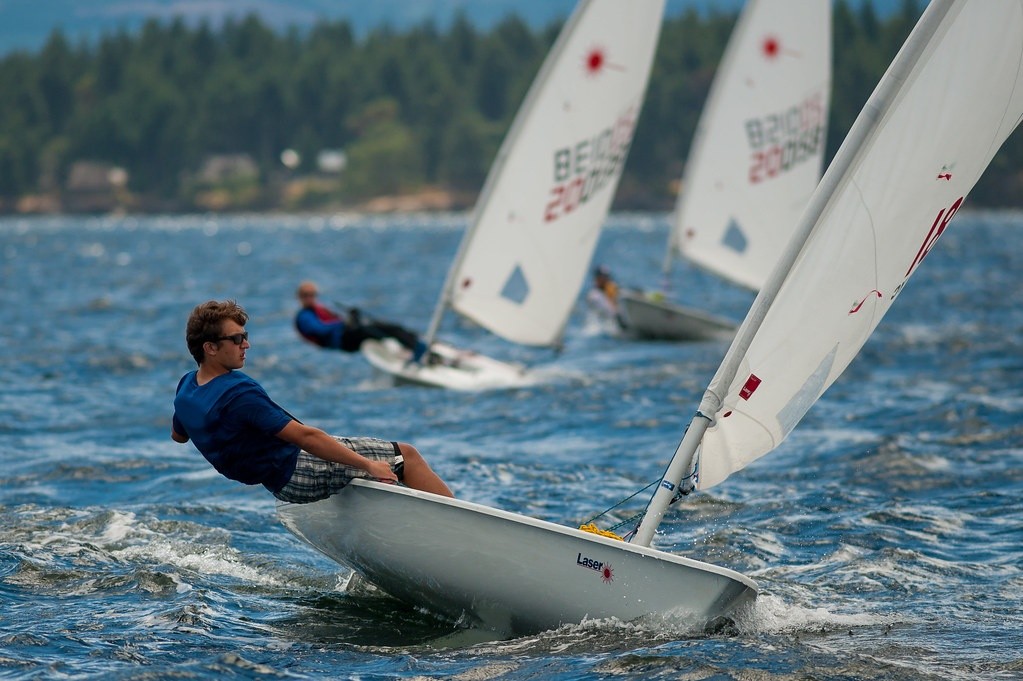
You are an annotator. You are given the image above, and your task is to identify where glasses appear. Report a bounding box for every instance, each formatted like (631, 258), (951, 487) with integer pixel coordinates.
(217, 331), (248, 345)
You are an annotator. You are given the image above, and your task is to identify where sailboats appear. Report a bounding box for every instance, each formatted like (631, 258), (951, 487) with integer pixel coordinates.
(588, 3), (839, 337)
(355, 1), (672, 397)
(272, 0), (1023, 612)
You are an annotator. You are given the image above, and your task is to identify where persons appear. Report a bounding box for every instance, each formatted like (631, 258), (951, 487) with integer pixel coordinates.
(593, 266), (617, 303)
(171, 299), (456, 504)
(289, 281), (429, 353)
